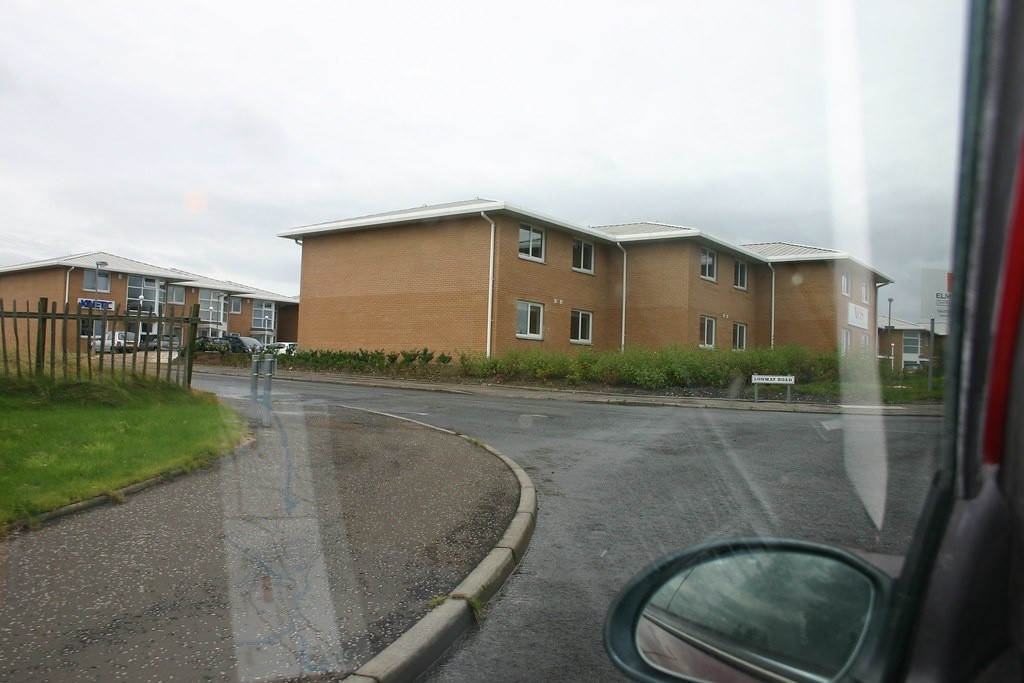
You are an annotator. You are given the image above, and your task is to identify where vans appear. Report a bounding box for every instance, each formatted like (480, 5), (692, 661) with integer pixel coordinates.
(264, 341), (297, 354)
(224, 336), (264, 353)
(194, 336), (232, 353)
(902, 363), (923, 377)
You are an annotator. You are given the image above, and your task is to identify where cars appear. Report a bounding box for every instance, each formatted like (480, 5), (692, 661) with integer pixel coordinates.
(92, 331), (141, 353)
(141, 335), (180, 351)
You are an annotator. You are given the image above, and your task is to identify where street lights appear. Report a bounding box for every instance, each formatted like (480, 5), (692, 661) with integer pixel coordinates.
(94, 261), (108, 335)
(217, 292), (224, 337)
(888, 297), (894, 358)
